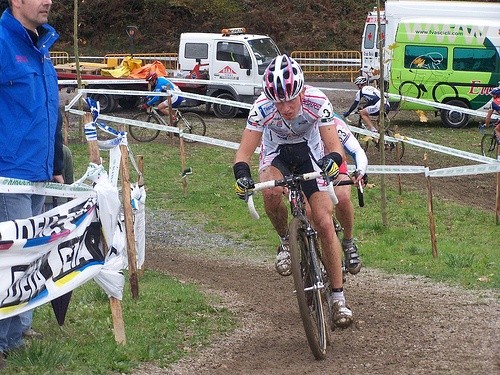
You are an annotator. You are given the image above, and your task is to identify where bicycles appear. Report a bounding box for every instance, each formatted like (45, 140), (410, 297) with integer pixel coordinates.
(345, 111), (405, 162)
(128, 97), (206, 144)
(244, 160), (364, 360)
(479, 119), (499, 159)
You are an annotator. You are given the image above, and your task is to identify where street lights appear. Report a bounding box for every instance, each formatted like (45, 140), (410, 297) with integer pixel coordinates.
(126, 26), (139, 59)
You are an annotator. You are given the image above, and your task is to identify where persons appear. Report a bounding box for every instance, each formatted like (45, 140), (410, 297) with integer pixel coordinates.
(138, 72), (184, 135)
(484, 87), (500, 139)
(285, 112), (368, 275)
(232, 53), (354, 330)
(47, 145), (75, 213)
(0, 0), (62, 361)
(341, 76), (390, 143)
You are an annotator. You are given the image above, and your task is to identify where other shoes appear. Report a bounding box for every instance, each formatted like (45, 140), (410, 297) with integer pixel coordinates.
(22, 328), (41, 337)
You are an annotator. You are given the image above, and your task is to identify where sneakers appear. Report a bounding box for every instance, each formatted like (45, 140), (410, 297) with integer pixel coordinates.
(332, 300), (353, 327)
(168, 116), (179, 126)
(342, 239), (362, 275)
(275, 234), (292, 276)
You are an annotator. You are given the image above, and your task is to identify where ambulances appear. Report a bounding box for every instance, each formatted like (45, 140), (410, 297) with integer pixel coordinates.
(360, 1), (500, 129)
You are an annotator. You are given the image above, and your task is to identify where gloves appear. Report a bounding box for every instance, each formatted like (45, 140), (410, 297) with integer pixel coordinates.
(233, 162), (255, 199)
(316, 152), (342, 181)
(138, 104), (148, 112)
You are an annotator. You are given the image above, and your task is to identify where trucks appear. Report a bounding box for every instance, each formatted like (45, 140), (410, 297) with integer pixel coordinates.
(57, 28), (282, 119)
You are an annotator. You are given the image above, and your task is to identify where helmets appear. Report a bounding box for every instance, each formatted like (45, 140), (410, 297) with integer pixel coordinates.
(354, 76), (366, 84)
(262, 54), (305, 103)
(490, 88), (500, 95)
(146, 71), (157, 81)
